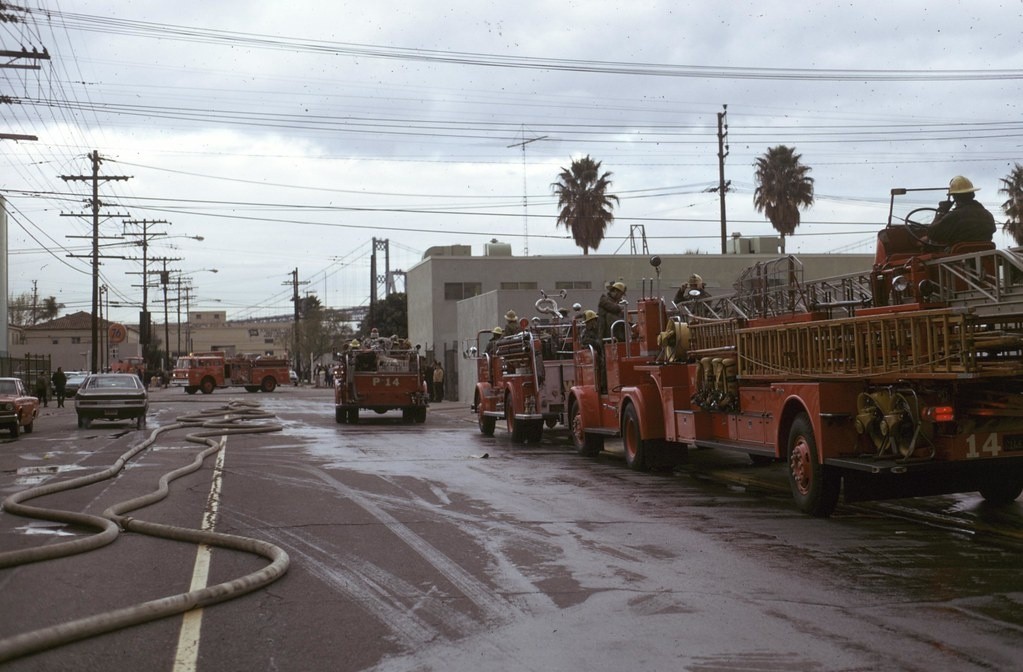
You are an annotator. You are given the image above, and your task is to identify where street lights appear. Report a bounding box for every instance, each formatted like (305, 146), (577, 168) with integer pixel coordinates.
(143, 235), (204, 385)
(185, 288), (221, 357)
(164, 269), (219, 389)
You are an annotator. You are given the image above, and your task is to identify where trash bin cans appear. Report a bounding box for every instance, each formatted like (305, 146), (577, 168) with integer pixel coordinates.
(151, 375), (163, 387)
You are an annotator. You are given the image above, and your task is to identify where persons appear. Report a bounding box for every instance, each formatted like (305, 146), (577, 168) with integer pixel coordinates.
(37, 371), (49, 407)
(426, 361), (444, 404)
(504, 310), (520, 336)
(314, 363), (334, 386)
(581, 309), (598, 348)
(595, 282), (627, 393)
(98, 367), (139, 374)
(350, 339), (360, 349)
(486, 327), (502, 353)
(674, 274), (712, 314)
(53, 367), (66, 408)
(926, 176), (997, 242)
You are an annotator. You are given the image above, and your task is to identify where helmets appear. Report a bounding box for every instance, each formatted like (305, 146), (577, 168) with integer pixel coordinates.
(946, 176), (981, 194)
(504, 310), (517, 320)
(606, 282), (627, 296)
(491, 327), (502, 334)
(350, 339), (360, 347)
(582, 310), (599, 323)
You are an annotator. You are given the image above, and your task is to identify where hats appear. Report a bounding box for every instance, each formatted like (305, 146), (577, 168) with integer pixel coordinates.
(688, 274), (703, 289)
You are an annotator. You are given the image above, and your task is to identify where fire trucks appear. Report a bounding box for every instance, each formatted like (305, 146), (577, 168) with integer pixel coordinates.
(545, 188), (1023, 519)
(171, 350), (291, 395)
(463, 289), (588, 444)
(332, 339), (431, 425)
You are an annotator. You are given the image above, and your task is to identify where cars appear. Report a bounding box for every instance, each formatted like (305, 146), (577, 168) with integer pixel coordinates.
(288, 370), (299, 386)
(0, 377), (40, 438)
(51, 371), (93, 395)
(74, 375), (148, 428)
(65, 374), (92, 398)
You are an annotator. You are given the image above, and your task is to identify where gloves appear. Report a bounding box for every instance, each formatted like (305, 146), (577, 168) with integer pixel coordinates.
(680, 282), (688, 292)
(939, 201), (954, 211)
